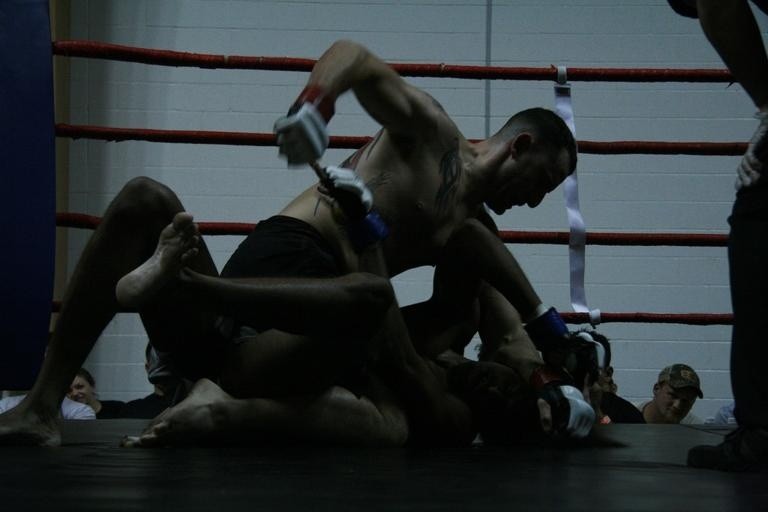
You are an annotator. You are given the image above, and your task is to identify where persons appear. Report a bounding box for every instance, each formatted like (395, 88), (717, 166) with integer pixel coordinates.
(635, 362), (704, 428)
(664, 0), (768, 474)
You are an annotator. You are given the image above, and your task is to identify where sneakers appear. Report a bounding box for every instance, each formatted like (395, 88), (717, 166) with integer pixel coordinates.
(687, 442), (724, 470)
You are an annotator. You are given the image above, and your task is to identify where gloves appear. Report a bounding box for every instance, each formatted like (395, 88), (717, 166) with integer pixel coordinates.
(704, 403), (735, 425)
(317, 167), (389, 255)
(522, 306), (577, 367)
(273, 84), (335, 165)
(736, 112), (767, 186)
(541, 386), (595, 439)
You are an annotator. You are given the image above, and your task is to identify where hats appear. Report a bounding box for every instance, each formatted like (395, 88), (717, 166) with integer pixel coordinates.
(659, 364), (703, 399)
